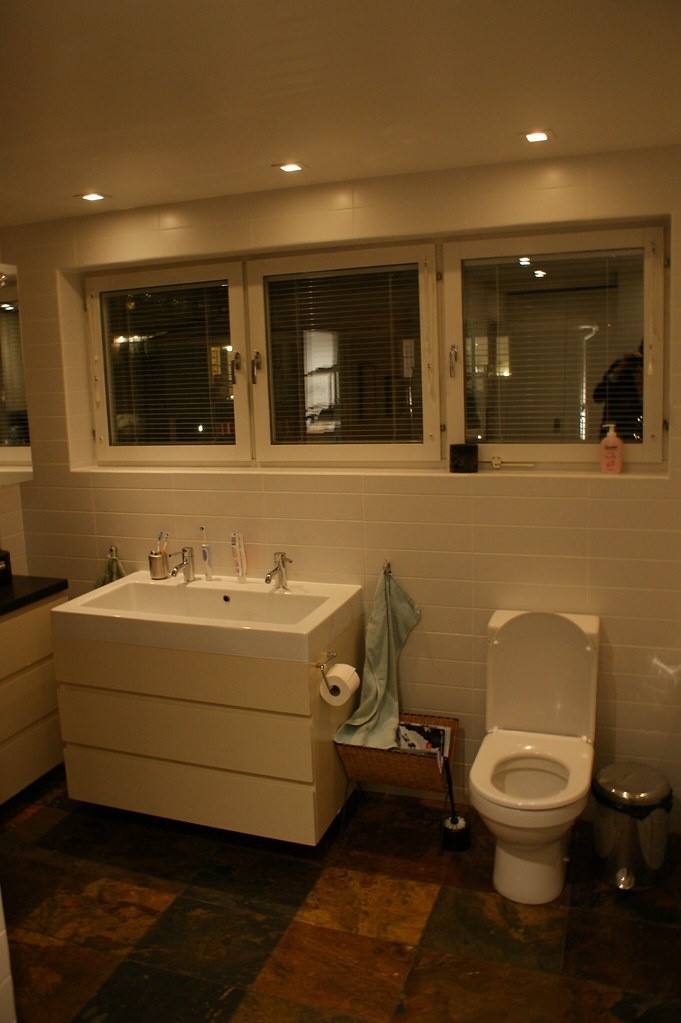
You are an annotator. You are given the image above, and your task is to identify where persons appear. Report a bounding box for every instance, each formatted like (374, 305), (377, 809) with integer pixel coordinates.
(593, 339), (643, 444)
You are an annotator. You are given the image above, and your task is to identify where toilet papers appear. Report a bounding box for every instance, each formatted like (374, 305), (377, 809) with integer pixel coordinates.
(319, 661), (360, 707)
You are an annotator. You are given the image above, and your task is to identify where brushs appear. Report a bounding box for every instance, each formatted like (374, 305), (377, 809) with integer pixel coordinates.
(438, 757), (472, 852)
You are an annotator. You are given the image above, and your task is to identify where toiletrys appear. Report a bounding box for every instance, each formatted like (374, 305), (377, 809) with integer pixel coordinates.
(229, 532), (249, 584)
(599, 422), (624, 473)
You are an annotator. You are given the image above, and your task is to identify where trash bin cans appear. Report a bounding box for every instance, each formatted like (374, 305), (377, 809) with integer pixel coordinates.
(591, 762), (673, 896)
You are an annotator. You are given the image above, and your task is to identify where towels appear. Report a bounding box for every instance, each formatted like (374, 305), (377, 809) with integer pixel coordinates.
(332, 569), (422, 750)
(90, 556), (126, 594)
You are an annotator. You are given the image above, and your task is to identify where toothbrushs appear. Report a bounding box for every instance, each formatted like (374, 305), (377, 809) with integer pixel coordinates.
(199, 525), (212, 581)
(156, 531), (162, 554)
(162, 532), (169, 554)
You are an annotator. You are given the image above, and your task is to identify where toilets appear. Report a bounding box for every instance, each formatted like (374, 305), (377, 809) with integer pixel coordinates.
(468, 609), (601, 906)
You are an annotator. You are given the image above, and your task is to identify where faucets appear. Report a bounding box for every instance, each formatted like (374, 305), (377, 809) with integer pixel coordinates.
(265, 550), (294, 590)
(168, 544), (196, 582)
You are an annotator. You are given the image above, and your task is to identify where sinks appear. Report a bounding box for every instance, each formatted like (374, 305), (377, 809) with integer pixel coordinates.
(76, 567), (330, 636)
